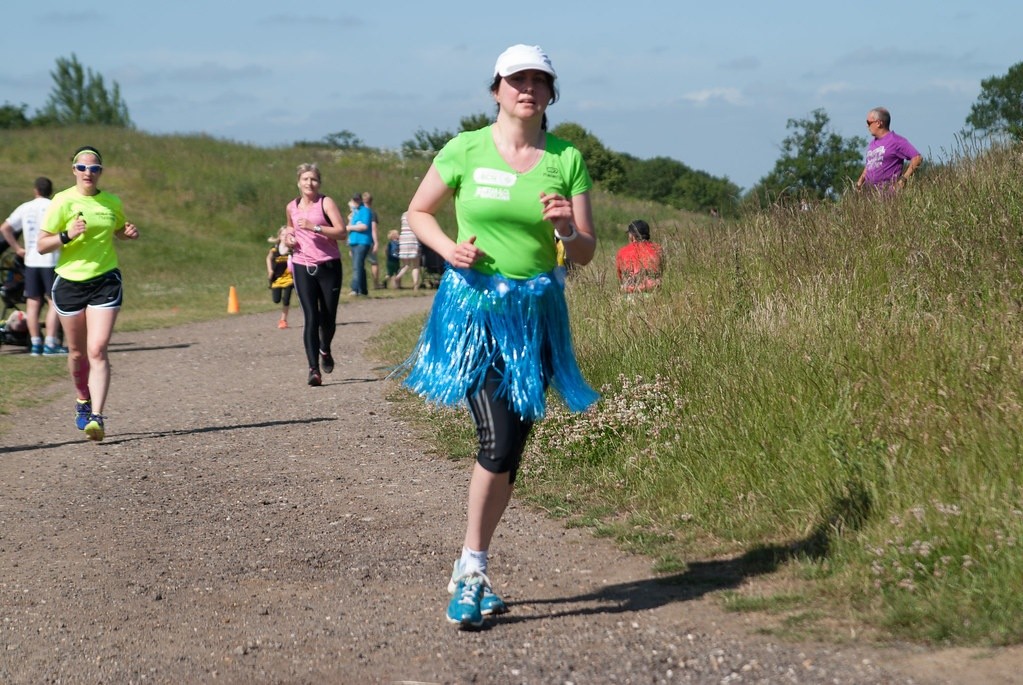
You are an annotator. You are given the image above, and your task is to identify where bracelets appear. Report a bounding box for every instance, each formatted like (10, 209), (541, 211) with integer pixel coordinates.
(900, 176), (908, 182)
(60, 231), (73, 244)
(554, 224), (578, 242)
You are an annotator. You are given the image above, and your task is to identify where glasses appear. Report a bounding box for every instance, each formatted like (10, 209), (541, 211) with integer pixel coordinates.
(865, 119), (884, 128)
(72, 163), (103, 174)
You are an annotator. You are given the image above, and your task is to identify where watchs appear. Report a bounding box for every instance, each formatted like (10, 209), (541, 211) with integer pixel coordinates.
(314, 224), (322, 233)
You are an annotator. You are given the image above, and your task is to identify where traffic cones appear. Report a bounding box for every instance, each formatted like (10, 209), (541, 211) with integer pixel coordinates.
(227, 285), (240, 314)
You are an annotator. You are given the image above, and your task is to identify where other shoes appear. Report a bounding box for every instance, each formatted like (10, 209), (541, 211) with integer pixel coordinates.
(0, 320), (8, 332)
(391, 276), (401, 289)
(358, 292), (367, 297)
(348, 289), (358, 296)
(382, 278), (388, 289)
(374, 285), (385, 289)
(413, 290), (426, 297)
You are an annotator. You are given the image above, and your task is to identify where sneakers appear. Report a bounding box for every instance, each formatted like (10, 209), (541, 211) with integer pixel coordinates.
(42, 343), (69, 355)
(447, 559), (505, 615)
(319, 347), (334, 374)
(447, 563), (488, 626)
(278, 319), (288, 329)
(30, 345), (42, 355)
(84, 414), (108, 441)
(307, 365), (322, 386)
(75, 398), (92, 431)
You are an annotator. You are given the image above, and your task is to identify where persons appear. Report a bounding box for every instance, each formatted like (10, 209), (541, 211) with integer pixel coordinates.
(407, 45), (596, 626)
(616, 220), (665, 292)
(266, 226), (293, 328)
(0, 177), (69, 355)
(382, 230), (402, 289)
(393, 211), (421, 290)
(284, 165), (348, 384)
(346, 192), (382, 295)
(855, 106), (923, 200)
(37, 146), (139, 440)
(799, 197), (810, 211)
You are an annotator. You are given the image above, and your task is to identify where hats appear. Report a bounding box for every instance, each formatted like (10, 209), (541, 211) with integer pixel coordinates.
(624, 219), (649, 235)
(493, 44), (557, 79)
